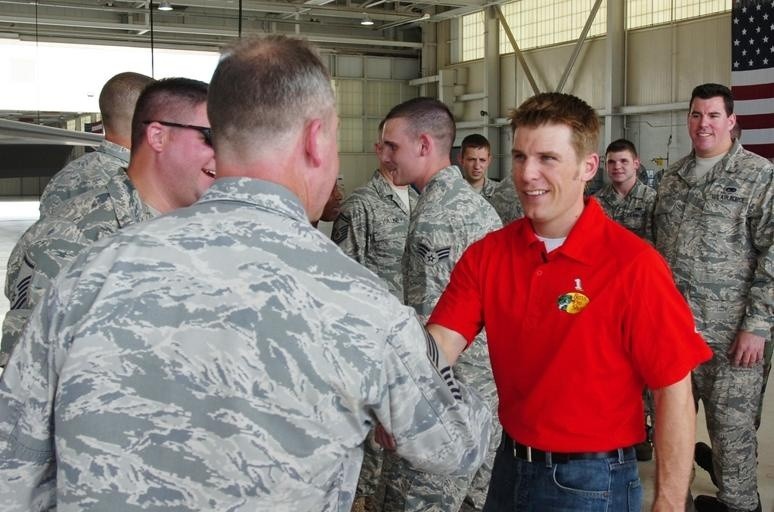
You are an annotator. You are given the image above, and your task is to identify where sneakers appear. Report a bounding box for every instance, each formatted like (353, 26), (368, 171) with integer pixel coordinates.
(692, 438), (722, 489)
(694, 491), (763, 512)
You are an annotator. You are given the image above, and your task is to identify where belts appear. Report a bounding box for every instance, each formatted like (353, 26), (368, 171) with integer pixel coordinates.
(499, 429), (635, 465)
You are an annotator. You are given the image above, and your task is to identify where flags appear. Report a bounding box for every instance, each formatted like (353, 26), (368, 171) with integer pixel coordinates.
(732, 0), (774, 162)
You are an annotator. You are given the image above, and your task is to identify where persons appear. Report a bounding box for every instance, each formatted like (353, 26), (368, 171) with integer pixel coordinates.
(489, 172), (525, 228)
(376, 92), (714, 512)
(654, 83), (774, 511)
(592, 139), (656, 246)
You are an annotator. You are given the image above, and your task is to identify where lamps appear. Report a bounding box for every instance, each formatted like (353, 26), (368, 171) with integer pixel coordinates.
(361, 14), (374, 25)
(157, 1), (173, 11)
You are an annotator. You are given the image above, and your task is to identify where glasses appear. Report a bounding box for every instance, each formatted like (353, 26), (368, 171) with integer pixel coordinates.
(143, 120), (213, 151)
